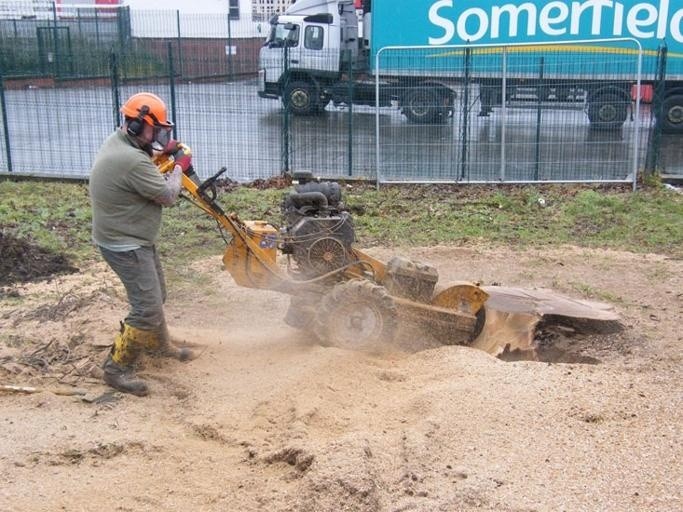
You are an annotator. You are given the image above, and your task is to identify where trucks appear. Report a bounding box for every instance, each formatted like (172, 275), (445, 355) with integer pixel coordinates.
(256, 0), (683, 132)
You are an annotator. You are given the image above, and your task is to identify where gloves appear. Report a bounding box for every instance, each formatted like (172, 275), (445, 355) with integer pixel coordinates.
(174, 155), (192, 173)
(163, 140), (178, 154)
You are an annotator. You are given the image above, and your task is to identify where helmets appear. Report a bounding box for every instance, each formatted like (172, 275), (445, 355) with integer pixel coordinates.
(118, 92), (175, 151)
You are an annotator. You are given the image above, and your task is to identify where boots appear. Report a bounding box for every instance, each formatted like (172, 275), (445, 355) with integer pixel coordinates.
(143, 322), (195, 360)
(103, 320), (161, 397)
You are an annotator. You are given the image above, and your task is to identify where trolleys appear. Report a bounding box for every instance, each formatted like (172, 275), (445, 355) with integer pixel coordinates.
(154, 139), (488, 353)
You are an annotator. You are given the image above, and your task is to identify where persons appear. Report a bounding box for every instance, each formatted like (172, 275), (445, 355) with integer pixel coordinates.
(89, 93), (200, 397)
